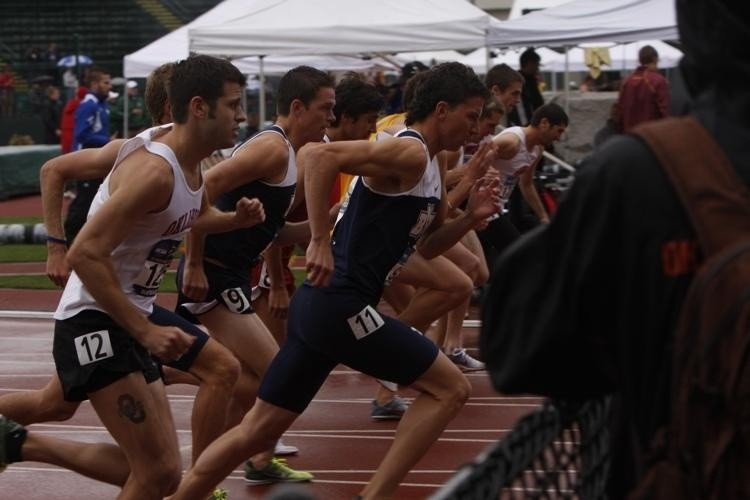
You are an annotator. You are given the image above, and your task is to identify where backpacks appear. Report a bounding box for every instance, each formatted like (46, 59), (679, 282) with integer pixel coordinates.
(634, 115), (749, 499)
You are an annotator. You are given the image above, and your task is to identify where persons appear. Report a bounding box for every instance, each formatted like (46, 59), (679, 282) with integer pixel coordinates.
(483, 1), (747, 498)
(1, 44), (665, 500)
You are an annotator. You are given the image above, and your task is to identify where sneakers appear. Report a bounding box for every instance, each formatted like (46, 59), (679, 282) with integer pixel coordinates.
(439, 350), (489, 372)
(275, 435), (301, 457)
(242, 460), (313, 484)
(0, 413), (28, 473)
(369, 397), (408, 418)
